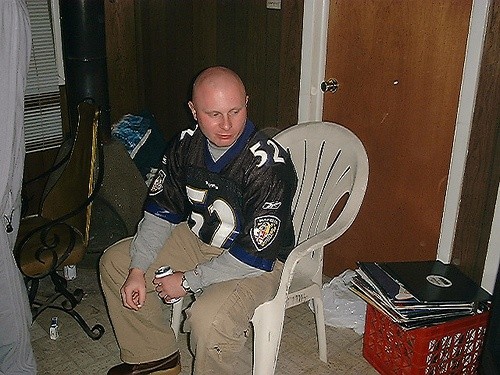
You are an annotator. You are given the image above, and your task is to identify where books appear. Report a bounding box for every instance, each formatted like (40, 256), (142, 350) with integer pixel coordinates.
(347, 260), (494, 330)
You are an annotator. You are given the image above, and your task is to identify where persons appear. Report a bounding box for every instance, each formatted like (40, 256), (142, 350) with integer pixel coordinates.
(98, 66), (299, 375)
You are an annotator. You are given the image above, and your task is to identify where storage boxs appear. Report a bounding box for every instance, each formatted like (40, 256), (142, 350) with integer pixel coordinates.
(362, 302), (490, 375)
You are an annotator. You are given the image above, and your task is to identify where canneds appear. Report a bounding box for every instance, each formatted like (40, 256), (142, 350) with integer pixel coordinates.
(155, 266), (182, 304)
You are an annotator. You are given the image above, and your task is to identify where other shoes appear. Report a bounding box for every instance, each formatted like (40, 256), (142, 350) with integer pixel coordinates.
(106, 350), (181, 375)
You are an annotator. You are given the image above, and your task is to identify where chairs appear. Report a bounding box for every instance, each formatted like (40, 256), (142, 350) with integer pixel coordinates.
(13, 98), (105, 340)
(171, 122), (370, 375)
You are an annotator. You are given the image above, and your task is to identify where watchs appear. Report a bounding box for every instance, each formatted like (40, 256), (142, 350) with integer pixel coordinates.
(181, 272), (194, 293)
